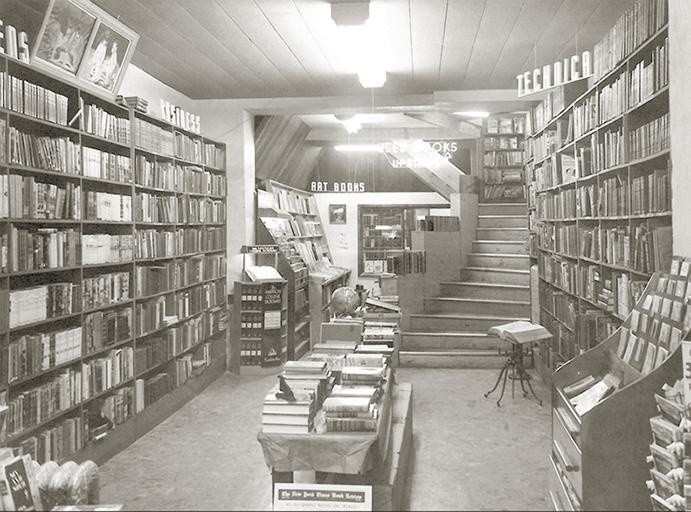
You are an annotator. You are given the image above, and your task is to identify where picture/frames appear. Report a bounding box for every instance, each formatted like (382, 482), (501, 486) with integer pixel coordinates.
(29, 0), (141, 102)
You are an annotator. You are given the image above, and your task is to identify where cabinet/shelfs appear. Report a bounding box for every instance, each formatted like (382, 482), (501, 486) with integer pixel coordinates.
(1, 52), (226, 468)
(230, 178), (352, 377)
(480, 113), (525, 202)
(358, 203), (450, 278)
(525, 0), (691, 511)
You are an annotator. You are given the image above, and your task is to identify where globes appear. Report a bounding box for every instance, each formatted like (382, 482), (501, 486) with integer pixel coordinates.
(329, 287), (360, 317)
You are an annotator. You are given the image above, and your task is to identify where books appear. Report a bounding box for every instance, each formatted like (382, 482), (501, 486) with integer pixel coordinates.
(129, 96), (226, 405)
(483, 118), (526, 199)
(261, 297), (401, 433)
(278, 190), (322, 267)
(1, 76), (134, 464)
(535, 0), (691, 512)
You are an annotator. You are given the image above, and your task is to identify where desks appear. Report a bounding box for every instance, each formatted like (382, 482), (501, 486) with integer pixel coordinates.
(484, 334), (551, 406)
